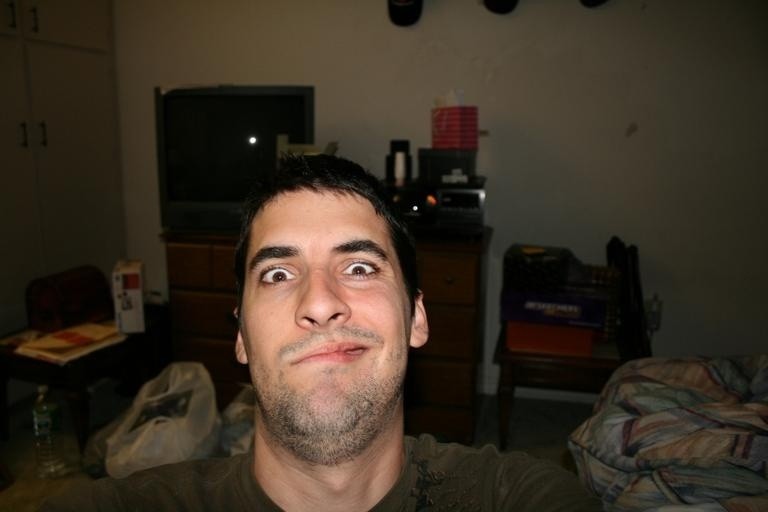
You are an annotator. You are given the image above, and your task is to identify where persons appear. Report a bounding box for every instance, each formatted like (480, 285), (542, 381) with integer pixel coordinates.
(1, 154), (606, 512)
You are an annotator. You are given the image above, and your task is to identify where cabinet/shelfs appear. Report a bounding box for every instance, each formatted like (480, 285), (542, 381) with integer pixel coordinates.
(160, 228), (494, 446)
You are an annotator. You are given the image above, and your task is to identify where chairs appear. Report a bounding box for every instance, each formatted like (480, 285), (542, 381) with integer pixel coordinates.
(23, 264), (114, 330)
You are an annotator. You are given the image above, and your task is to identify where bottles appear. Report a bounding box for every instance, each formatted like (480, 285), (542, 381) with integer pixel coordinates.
(33, 384), (65, 479)
(648, 301), (659, 324)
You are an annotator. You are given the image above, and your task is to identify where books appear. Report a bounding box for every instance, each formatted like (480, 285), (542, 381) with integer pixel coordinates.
(19, 323), (127, 361)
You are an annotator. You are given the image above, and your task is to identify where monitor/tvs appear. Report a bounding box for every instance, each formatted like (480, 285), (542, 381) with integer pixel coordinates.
(153, 82), (315, 237)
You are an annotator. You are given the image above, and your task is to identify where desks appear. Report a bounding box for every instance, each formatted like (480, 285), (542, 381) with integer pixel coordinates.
(1, 322), (133, 462)
(493, 318), (625, 448)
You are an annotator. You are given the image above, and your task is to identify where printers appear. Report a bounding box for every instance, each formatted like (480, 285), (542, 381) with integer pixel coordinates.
(499, 242), (620, 333)
